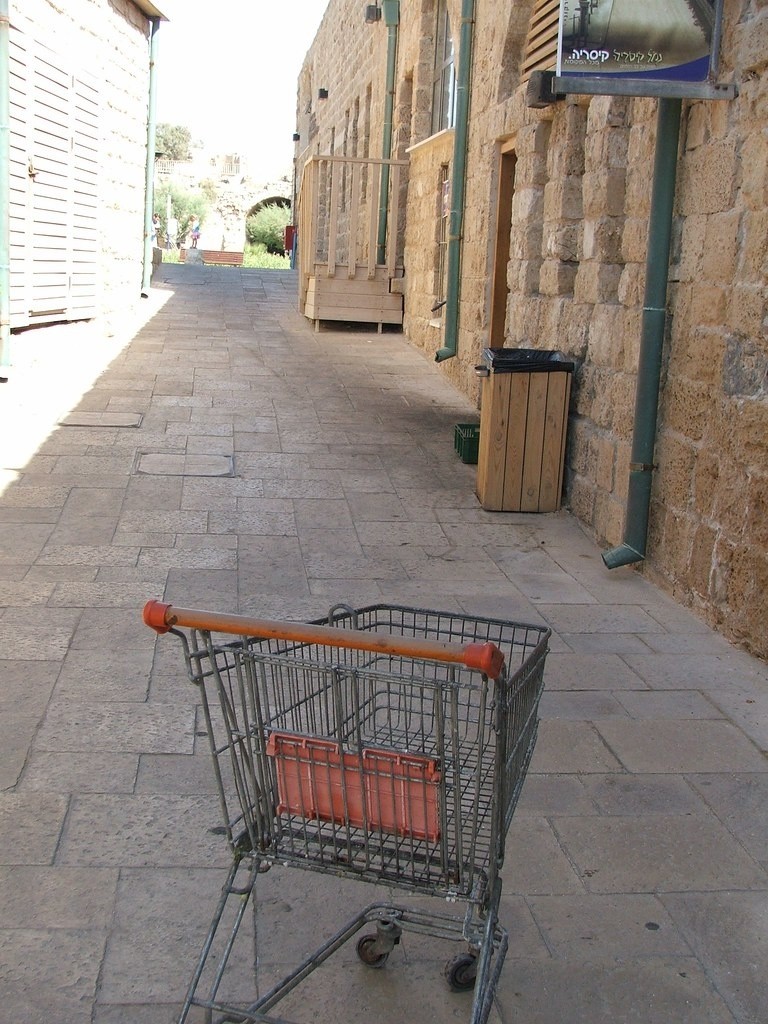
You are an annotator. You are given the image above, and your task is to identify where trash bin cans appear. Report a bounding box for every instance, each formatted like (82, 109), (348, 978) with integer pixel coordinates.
(474, 348), (575, 513)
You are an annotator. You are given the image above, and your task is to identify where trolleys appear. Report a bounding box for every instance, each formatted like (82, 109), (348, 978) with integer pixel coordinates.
(144, 598), (553, 1024)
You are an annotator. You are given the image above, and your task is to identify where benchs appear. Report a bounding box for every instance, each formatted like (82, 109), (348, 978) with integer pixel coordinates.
(178, 249), (244, 268)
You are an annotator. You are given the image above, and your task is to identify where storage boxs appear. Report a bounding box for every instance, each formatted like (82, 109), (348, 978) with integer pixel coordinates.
(453, 422), (480, 465)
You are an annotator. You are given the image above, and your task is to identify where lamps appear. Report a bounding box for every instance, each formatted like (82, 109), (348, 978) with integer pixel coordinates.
(317, 89), (328, 100)
(366, 5), (381, 26)
(526, 70), (567, 110)
(293, 134), (300, 142)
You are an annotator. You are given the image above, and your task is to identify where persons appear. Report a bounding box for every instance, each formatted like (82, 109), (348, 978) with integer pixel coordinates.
(151, 213), (162, 262)
(190, 214), (201, 249)
